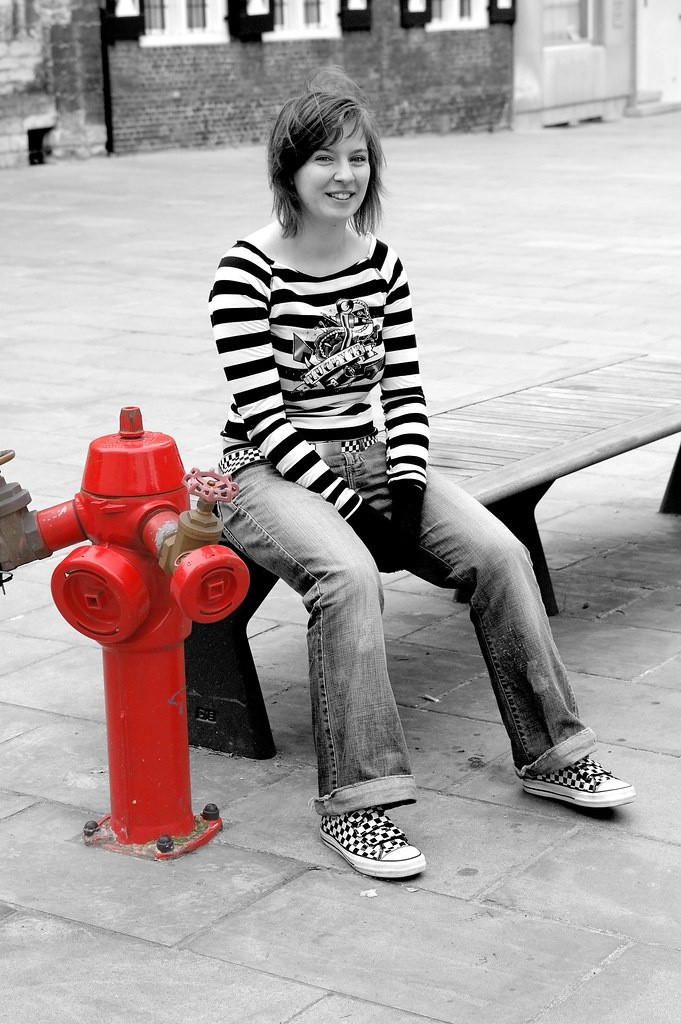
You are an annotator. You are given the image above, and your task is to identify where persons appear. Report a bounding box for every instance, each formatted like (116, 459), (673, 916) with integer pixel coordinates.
(206, 91), (637, 879)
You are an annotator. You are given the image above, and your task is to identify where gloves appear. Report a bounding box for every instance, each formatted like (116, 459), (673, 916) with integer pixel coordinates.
(388, 484), (424, 541)
(346, 502), (415, 573)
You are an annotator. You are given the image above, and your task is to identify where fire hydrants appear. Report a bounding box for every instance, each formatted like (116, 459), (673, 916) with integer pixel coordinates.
(0, 403), (251, 860)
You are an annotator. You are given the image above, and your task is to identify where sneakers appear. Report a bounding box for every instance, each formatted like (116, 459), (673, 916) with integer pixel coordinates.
(318, 808), (427, 878)
(524, 755), (637, 808)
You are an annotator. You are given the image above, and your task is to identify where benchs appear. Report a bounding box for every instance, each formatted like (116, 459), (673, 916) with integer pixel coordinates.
(183, 348), (681, 759)
